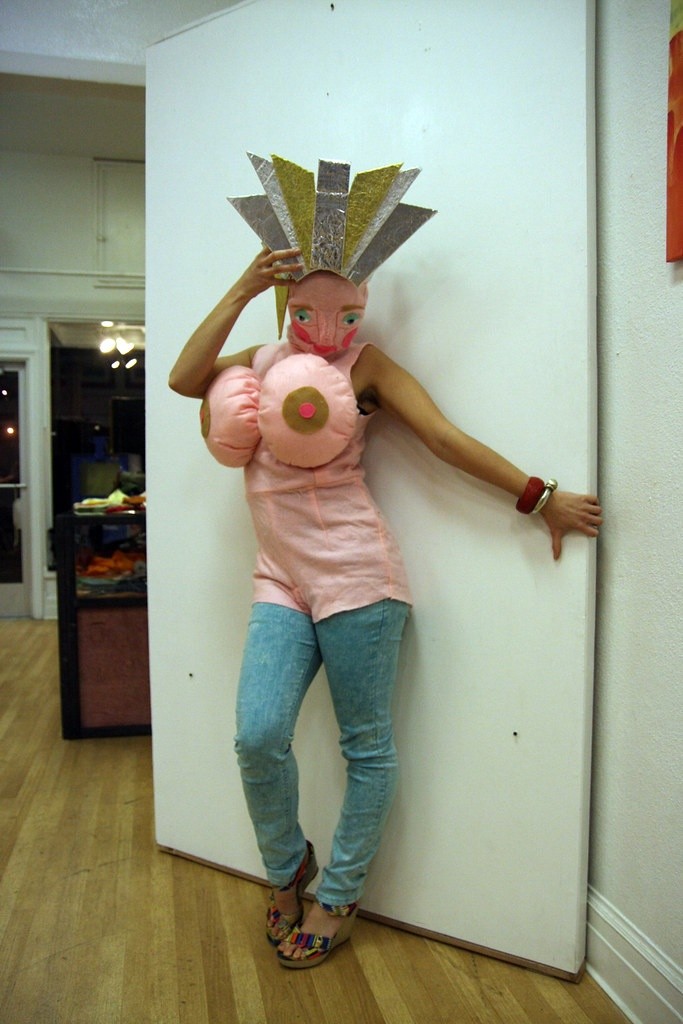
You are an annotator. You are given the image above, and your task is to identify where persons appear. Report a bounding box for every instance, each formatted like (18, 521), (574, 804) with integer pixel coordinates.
(169, 246), (602, 968)
(0, 440), (19, 553)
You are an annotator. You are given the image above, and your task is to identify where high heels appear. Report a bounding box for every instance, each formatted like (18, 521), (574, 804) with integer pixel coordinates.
(277, 900), (358, 968)
(266, 839), (319, 944)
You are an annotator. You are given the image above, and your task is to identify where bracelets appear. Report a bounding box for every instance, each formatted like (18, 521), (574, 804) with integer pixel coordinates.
(532, 479), (558, 514)
(516, 477), (544, 514)
